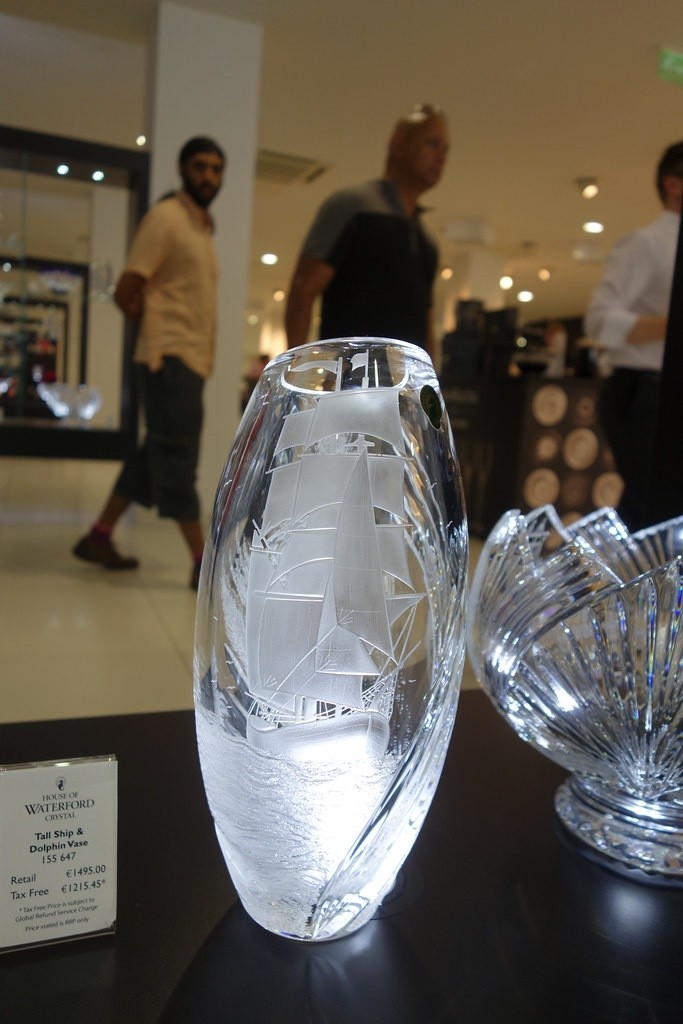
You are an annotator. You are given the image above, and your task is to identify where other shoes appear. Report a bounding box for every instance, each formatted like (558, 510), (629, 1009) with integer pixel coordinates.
(74, 532), (138, 569)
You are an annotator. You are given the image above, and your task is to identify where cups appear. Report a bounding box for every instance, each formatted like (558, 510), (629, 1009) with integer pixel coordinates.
(194, 338), (466, 941)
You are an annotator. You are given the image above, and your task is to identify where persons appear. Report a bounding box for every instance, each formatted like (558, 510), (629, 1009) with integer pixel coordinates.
(586, 141), (683, 532)
(72, 136), (226, 592)
(284, 104), (449, 354)
